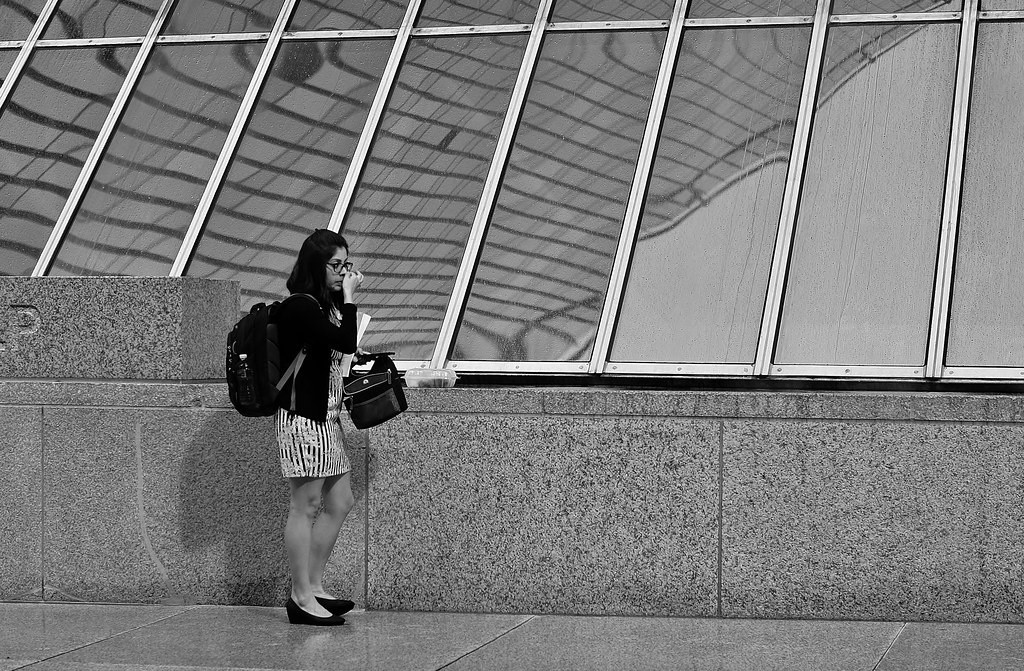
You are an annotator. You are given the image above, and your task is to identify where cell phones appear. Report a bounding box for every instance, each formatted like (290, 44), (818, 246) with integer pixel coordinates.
(356, 351), (395, 359)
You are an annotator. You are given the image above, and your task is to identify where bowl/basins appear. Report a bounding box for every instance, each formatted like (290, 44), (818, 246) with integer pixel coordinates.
(400, 368), (460, 388)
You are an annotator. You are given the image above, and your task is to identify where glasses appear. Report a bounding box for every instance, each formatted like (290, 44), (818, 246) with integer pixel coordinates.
(327, 262), (353, 273)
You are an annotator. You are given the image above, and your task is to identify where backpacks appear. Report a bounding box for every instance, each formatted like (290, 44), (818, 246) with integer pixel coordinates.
(226, 293), (326, 417)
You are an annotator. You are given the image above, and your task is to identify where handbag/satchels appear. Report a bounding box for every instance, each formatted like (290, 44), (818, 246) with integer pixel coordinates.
(342, 354), (410, 429)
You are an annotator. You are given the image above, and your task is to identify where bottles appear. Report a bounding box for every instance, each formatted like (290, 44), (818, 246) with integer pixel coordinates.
(235, 354), (252, 405)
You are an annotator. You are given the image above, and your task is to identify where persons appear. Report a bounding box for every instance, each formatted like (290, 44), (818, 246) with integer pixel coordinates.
(276, 229), (372, 626)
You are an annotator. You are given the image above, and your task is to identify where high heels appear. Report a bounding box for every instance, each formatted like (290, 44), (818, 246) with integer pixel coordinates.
(314, 596), (355, 616)
(287, 598), (345, 626)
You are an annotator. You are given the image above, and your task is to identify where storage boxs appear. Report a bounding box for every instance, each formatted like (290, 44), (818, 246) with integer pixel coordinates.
(401, 368), (460, 388)
